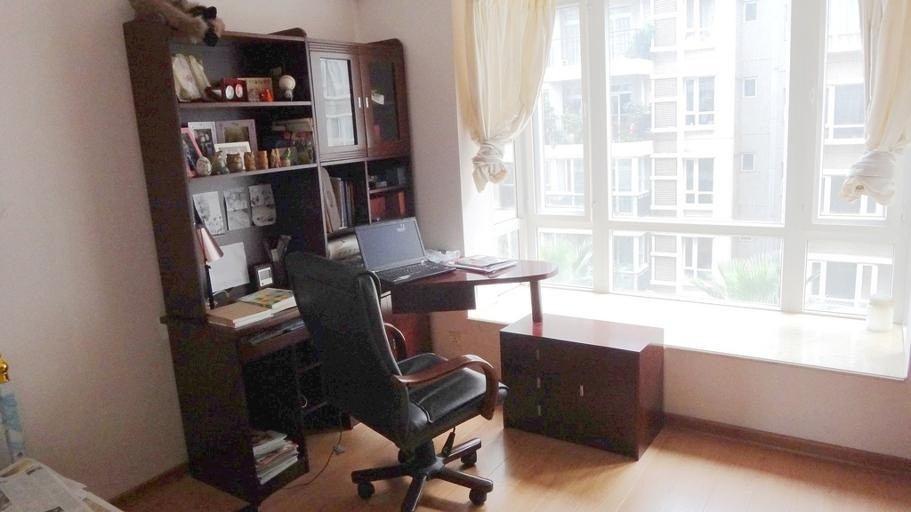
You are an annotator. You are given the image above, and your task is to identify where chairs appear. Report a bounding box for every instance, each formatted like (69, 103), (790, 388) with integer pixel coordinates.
(286, 250), (508, 511)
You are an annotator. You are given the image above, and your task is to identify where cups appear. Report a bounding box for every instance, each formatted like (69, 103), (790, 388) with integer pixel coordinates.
(244, 150), (269, 171)
(226, 153), (243, 173)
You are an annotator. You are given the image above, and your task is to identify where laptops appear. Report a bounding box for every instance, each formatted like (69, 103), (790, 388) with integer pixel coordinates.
(353, 216), (456, 288)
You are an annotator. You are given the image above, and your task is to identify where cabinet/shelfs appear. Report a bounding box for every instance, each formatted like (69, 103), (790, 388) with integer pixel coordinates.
(500, 313), (666, 460)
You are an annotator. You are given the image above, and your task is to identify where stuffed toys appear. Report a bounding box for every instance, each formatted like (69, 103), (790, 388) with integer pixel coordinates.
(127, 0), (228, 48)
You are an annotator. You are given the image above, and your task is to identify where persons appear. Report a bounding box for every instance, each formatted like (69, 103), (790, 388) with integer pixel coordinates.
(182, 136), (197, 171)
(205, 132), (214, 157)
(197, 132), (208, 158)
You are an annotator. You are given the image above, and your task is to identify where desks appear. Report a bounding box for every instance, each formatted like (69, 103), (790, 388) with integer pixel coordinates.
(383, 256), (559, 322)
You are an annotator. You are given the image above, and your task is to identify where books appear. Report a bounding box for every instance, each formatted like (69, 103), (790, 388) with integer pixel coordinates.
(250, 427), (302, 487)
(445, 256), (520, 273)
(455, 254), (515, 270)
(320, 167), (357, 234)
(206, 285), (299, 330)
